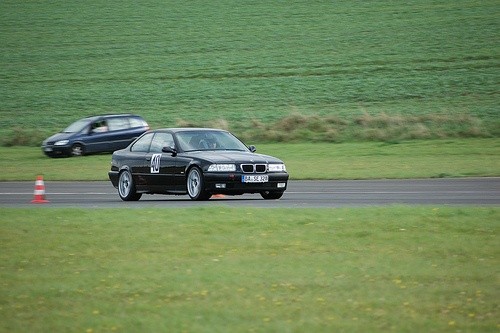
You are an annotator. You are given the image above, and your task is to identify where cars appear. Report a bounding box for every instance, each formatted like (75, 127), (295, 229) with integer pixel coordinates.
(109, 127), (290, 203)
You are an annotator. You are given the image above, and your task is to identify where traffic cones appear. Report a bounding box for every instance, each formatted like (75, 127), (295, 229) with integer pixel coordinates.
(31, 175), (48, 203)
(213, 195), (224, 199)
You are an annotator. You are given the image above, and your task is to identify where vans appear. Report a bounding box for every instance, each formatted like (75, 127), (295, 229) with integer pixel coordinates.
(41, 116), (151, 157)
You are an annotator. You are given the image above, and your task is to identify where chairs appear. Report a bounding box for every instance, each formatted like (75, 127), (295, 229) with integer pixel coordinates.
(189, 135), (209, 148)
